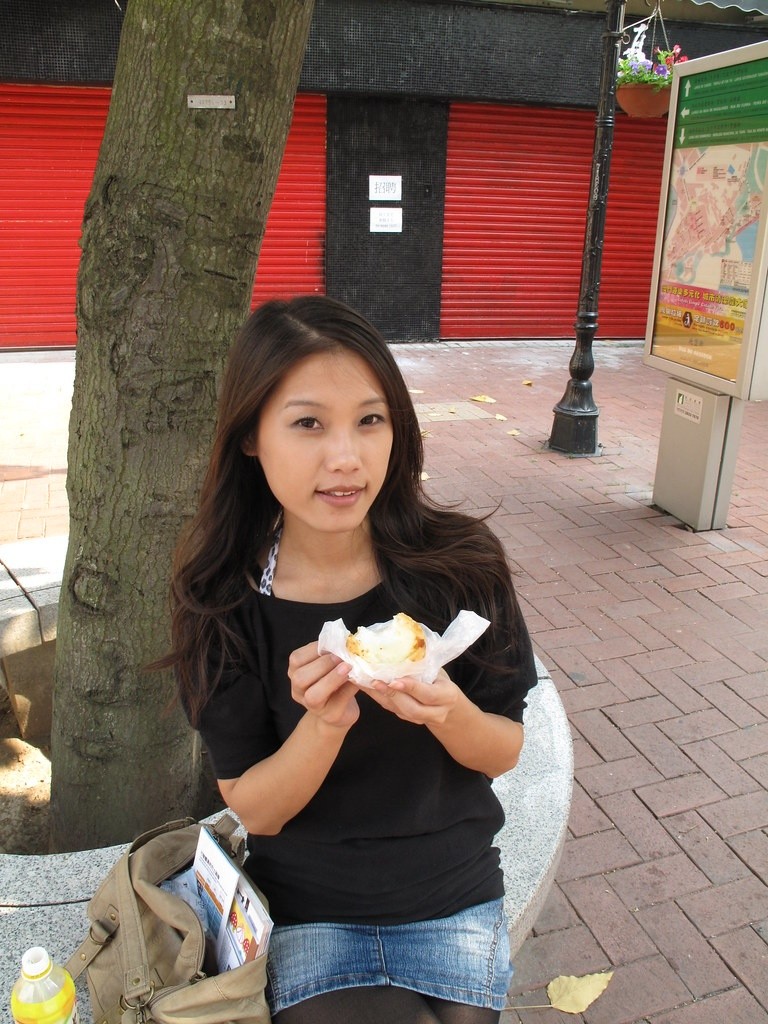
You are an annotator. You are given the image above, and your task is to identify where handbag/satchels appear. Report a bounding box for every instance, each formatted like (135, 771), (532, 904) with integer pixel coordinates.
(63, 816), (272, 1024)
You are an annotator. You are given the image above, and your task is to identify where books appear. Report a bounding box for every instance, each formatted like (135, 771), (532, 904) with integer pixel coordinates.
(192, 826), (273, 973)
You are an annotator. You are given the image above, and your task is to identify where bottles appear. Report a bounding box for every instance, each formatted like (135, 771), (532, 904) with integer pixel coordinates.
(11, 947), (79, 1024)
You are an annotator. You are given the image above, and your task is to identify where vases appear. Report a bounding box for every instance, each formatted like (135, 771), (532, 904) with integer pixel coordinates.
(615, 84), (671, 118)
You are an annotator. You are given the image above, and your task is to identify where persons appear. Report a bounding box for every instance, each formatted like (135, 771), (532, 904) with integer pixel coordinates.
(168, 294), (538, 1024)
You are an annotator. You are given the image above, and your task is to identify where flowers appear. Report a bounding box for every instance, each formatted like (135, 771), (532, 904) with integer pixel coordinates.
(611, 45), (688, 94)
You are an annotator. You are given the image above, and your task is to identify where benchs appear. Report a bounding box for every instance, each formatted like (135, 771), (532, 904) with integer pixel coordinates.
(0, 533), (576, 1024)
